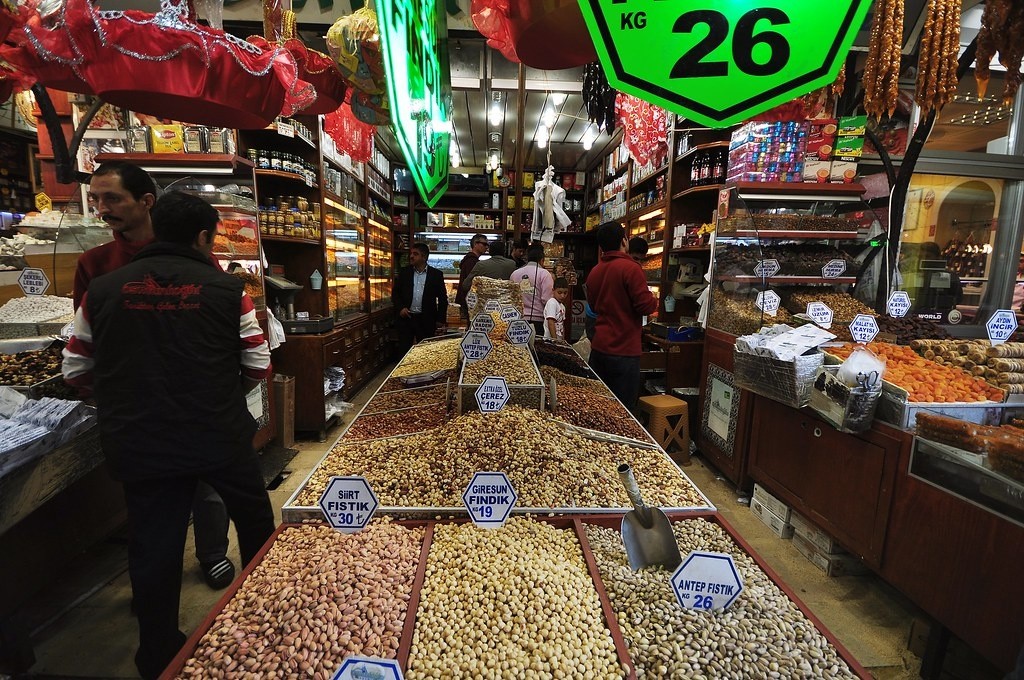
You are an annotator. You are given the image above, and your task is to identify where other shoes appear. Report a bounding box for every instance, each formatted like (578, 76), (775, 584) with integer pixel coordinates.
(200, 557), (235, 590)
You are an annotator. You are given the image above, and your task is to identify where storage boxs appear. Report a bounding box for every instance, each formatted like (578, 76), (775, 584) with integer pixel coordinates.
(202, 127), (231, 155)
(986, 136), (1008, 155)
(322, 130), (365, 206)
(0, 405), (106, 535)
(7, 371), (80, 402)
(183, 127), (205, 153)
(585, 142), (628, 232)
(670, 281), (686, 300)
(38, 313), (68, 336)
(677, 264), (703, 284)
(673, 227), (678, 238)
(673, 240), (676, 250)
(416, 171), (585, 274)
(669, 253), (680, 266)
(802, 160), (831, 183)
(836, 115), (867, 137)
(149, 124), (186, 154)
(831, 136), (864, 156)
(675, 236), (682, 249)
(830, 161), (858, 184)
(629, 158), (668, 212)
(678, 225), (687, 237)
(127, 126), (152, 153)
(804, 136), (834, 161)
(806, 119), (838, 137)
(154, 317), (1024, 680)
(0, 312), (66, 340)
(832, 155), (861, 163)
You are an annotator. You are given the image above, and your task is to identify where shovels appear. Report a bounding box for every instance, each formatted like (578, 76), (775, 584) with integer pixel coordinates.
(617, 463), (682, 570)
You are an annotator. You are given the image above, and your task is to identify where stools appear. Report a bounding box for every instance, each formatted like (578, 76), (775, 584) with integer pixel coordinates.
(635, 395), (692, 467)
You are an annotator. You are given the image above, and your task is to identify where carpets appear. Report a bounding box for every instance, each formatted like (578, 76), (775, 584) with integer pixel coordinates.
(256, 445), (299, 486)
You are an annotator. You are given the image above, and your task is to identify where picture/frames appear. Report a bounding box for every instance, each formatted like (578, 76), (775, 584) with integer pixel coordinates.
(903, 188), (923, 232)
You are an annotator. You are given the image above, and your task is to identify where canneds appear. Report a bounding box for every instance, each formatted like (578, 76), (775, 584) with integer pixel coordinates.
(247, 149), (316, 182)
(259, 196), (321, 240)
(274, 116), (312, 141)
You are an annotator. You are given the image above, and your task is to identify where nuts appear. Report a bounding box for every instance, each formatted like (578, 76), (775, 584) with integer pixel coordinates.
(291, 335), (709, 507)
(403, 513), (630, 680)
(582, 517), (860, 680)
(175, 514), (426, 680)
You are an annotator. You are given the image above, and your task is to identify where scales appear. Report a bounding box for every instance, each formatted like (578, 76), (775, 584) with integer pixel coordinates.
(263, 274), (334, 334)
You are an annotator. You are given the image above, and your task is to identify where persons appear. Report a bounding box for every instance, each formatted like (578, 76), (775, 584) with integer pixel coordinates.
(585, 221), (657, 414)
(62, 159), (276, 680)
(916, 242), (963, 309)
(391, 242), (448, 360)
(455, 233), (569, 340)
(1011, 254), (1024, 309)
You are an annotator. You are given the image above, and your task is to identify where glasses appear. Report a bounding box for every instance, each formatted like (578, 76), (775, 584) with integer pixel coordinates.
(476, 242), (489, 246)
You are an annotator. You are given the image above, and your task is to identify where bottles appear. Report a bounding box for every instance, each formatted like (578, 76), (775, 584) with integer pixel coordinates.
(629, 191), (647, 213)
(274, 297), (281, 316)
(689, 150), (725, 188)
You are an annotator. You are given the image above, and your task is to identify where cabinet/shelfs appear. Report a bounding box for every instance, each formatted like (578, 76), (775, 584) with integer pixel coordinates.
(245, 115), (400, 453)
(690, 182), (866, 497)
(581, 114), (731, 425)
(93, 151), (278, 454)
(503, 167), (586, 342)
(0, 224), (84, 308)
(390, 158), (416, 361)
(31, 87), (84, 215)
(410, 174), (506, 337)
